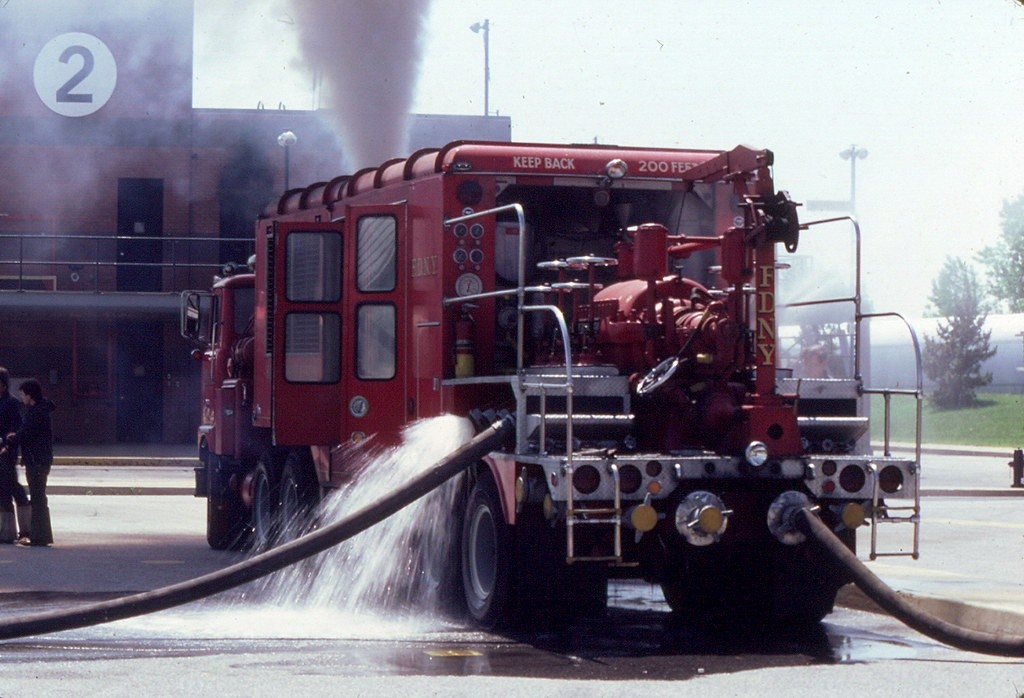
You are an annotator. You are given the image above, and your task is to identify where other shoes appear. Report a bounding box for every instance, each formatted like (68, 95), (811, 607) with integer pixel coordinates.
(19, 532), (51, 547)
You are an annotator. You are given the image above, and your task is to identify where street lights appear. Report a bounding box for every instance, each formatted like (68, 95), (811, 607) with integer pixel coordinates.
(837, 143), (868, 214)
(275, 130), (298, 197)
(468, 19), (493, 115)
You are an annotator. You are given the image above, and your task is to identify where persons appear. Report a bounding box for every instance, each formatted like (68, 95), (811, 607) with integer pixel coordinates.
(573, 188), (633, 232)
(0, 366), (57, 547)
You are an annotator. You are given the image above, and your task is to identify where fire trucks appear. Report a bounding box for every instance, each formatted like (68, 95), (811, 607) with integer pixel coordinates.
(173, 139), (926, 651)
